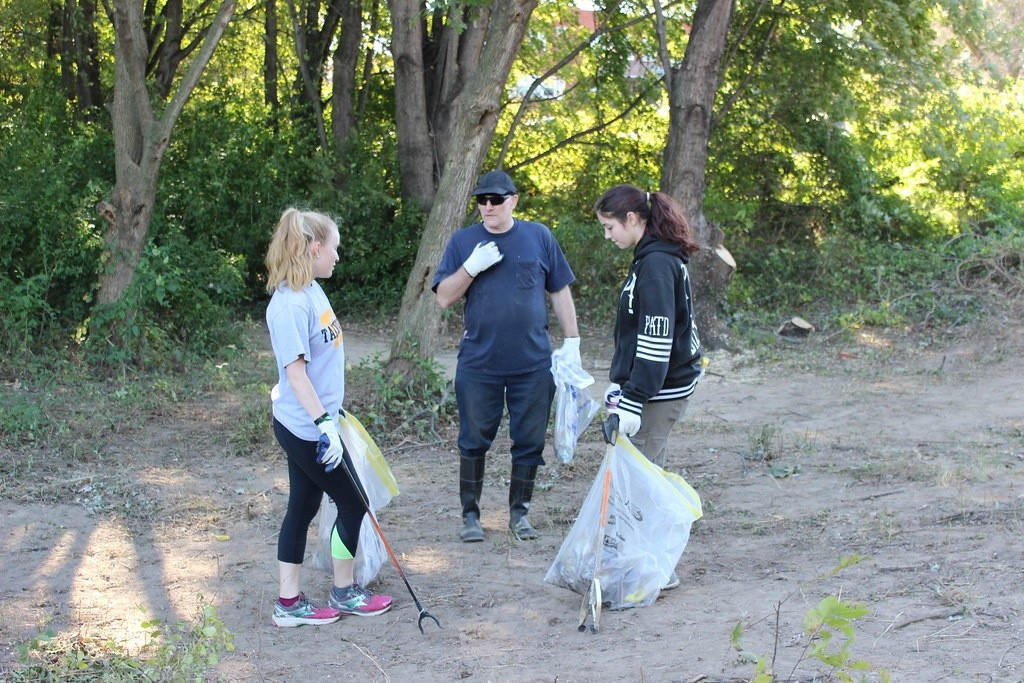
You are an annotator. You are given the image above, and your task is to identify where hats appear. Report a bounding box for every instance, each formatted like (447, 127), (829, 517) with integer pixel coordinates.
(473, 170), (516, 194)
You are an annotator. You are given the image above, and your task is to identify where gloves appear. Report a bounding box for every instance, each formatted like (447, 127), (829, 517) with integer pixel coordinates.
(560, 336), (582, 366)
(464, 240), (504, 279)
(315, 411), (344, 473)
(604, 383), (620, 404)
(610, 408), (641, 437)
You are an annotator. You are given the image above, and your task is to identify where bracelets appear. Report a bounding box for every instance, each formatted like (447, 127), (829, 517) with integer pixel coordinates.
(314, 412), (331, 425)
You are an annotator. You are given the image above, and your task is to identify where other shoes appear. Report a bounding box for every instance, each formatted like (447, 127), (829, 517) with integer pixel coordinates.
(662, 572), (680, 589)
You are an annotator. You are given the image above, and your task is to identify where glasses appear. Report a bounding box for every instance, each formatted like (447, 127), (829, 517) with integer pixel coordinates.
(476, 196), (511, 205)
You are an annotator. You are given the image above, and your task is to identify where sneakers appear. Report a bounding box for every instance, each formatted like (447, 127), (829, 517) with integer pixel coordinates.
(329, 578), (392, 617)
(271, 591), (341, 628)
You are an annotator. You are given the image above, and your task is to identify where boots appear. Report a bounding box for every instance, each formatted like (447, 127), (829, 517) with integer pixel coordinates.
(459, 455), (486, 541)
(508, 462), (538, 539)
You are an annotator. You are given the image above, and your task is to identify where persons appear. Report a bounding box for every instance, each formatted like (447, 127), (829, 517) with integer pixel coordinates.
(431, 171), (581, 542)
(593, 185), (702, 590)
(263, 208), (393, 630)
